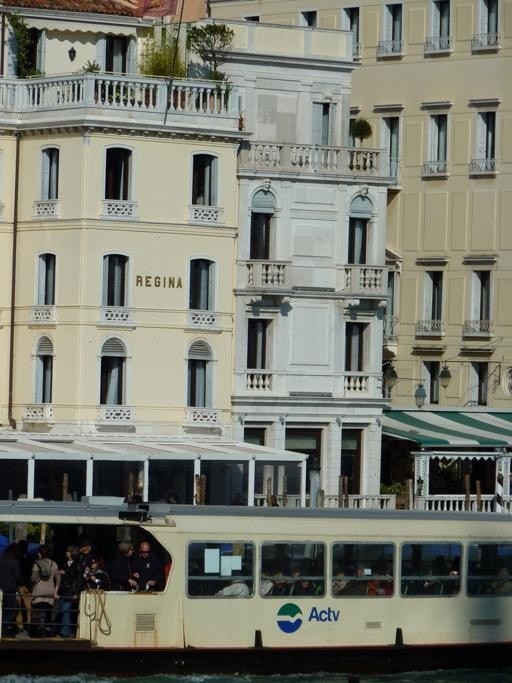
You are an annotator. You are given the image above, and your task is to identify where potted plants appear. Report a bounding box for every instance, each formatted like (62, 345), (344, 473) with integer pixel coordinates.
(197, 70), (229, 113)
(137, 39), (185, 107)
(72, 60), (104, 103)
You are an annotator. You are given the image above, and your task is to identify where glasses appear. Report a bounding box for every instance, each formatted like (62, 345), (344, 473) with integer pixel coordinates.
(90, 561), (96, 564)
(140, 551), (150, 553)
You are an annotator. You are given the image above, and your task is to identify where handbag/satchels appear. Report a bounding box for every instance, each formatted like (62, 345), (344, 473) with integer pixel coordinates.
(35, 558), (52, 576)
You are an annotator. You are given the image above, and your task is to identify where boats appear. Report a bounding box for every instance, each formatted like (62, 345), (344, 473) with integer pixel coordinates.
(0, 497), (511, 672)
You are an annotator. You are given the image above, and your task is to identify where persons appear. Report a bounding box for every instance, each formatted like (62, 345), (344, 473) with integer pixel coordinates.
(1, 540), (161, 638)
(213, 555), (512, 597)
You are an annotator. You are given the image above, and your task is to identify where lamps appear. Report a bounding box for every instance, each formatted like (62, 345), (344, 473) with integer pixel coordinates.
(67, 46), (76, 62)
(382, 361), (398, 388)
(417, 476), (423, 496)
(415, 377), (426, 412)
(439, 359), (452, 387)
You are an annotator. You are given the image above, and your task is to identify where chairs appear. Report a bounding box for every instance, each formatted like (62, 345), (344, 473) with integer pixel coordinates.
(429, 580), (444, 596)
(401, 583), (408, 596)
(283, 582), (295, 595)
